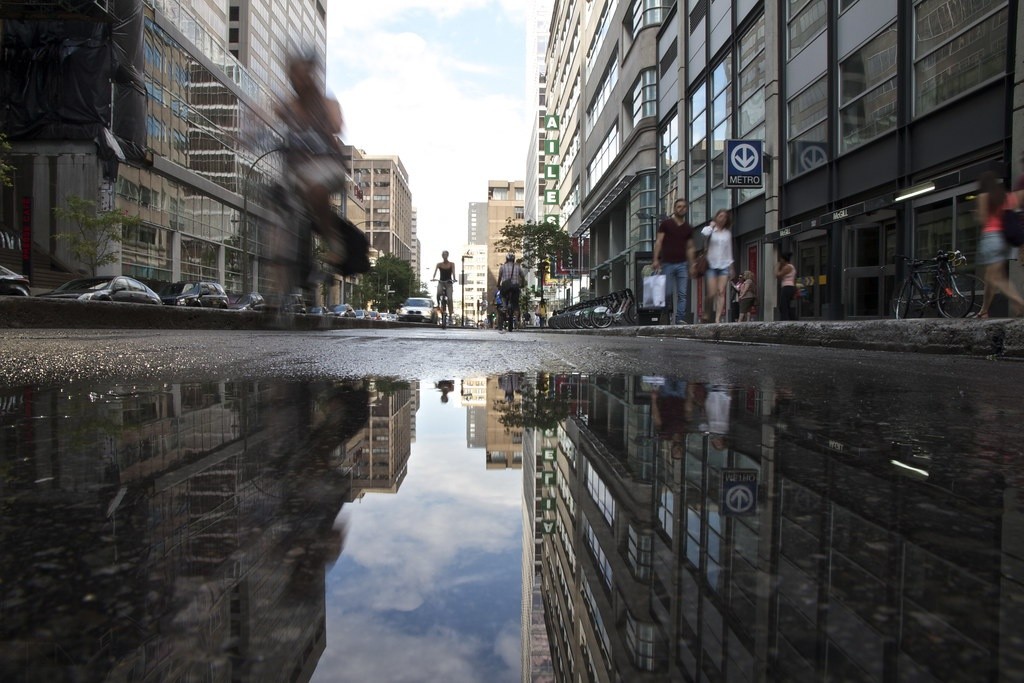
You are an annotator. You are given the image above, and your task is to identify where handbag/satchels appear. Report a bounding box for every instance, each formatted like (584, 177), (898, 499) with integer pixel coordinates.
(501, 279), (512, 295)
(642, 266), (666, 310)
(1002, 208), (1024, 248)
(689, 226), (714, 277)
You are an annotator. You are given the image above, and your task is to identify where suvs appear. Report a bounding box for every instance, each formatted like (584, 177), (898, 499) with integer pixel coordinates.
(158, 280), (230, 310)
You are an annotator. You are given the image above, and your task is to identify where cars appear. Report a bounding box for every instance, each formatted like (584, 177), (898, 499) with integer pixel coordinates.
(399, 296), (439, 326)
(310, 304), (398, 323)
(229, 292), (306, 312)
(0, 266), (31, 297)
(35, 275), (162, 303)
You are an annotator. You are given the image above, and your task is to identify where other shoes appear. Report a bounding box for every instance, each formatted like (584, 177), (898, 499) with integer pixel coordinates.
(660, 312), (671, 324)
(675, 319), (687, 324)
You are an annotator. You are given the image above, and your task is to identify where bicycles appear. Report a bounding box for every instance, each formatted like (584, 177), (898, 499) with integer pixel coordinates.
(432, 282), (456, 329)
(545, 288), (639, 330)
(491, 285), (530, 334)
(894, 249), (988, 321)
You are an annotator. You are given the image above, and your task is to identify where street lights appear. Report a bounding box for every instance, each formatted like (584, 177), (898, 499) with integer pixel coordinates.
(385, 250), (410, 314)
(231, 216), (258, 293)
(241, 146), (299, 305)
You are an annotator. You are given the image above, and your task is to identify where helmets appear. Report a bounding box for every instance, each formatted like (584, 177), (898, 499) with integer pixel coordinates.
(506, 253), (515, 261)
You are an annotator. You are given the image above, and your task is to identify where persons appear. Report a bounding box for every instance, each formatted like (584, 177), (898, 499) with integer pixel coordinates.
(731, 270), (755, 321)
(695, 383), (734, 450)
(498, 373), (523, 402)
(538, 299), (547, 328)
(651, 381), (696, 459)
(701, 209), (734, 322)
(497, 254), (525, 321)
(433, 251), (456, 325)
(266, 47), (374, 324)
(652, 199), (698, 324)
(974, 171), (1024, 318)
(437, 381), (455, 403)
(776, 252), (798, 320)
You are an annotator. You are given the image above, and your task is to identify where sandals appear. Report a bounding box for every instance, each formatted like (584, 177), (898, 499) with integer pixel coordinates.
(970, 312), (989, 319)
